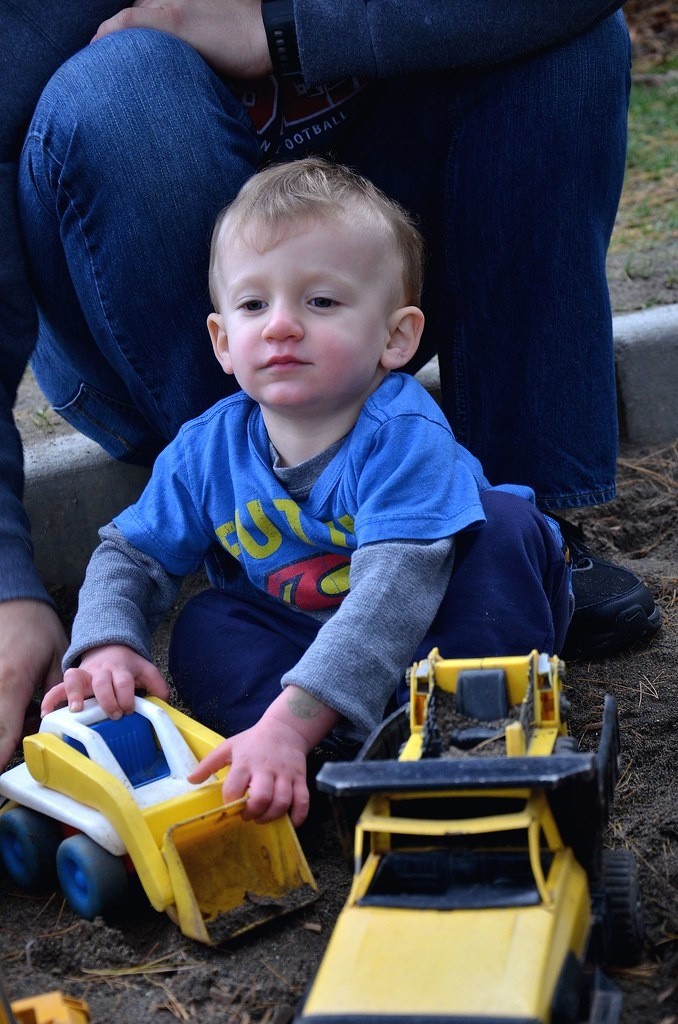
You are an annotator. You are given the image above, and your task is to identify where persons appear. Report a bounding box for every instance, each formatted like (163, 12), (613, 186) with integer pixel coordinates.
(0, 1), (657, 777)
(37, 153), (573, 844)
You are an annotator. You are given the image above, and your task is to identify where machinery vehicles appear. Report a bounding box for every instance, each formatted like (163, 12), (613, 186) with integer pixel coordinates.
(0, 697), (324, 953)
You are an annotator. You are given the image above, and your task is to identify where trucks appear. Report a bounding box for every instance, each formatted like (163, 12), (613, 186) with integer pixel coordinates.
(295, 651), (641, 1023)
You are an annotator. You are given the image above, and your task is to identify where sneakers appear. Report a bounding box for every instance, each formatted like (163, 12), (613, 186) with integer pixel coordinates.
(538, 509), (663, 653)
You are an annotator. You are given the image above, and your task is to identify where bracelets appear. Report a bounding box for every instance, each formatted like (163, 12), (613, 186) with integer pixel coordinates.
(259, 0), (302, 83)
